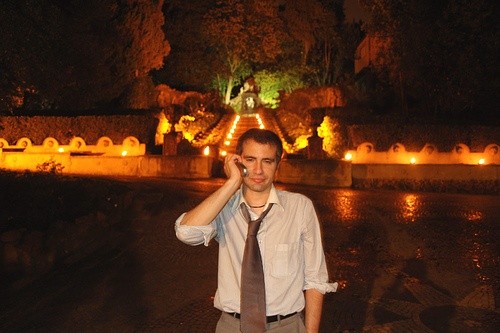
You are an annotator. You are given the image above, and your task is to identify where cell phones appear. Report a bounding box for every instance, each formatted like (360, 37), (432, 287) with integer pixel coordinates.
(235, 162), (248, 177)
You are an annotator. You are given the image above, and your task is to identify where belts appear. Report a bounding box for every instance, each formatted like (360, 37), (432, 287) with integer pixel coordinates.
(227, 312), (297, 323)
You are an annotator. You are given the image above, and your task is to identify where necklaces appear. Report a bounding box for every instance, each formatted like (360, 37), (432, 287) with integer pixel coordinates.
(247, 202), (267, 208)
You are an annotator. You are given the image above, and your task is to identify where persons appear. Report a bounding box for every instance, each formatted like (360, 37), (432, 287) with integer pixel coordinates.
(174, 126), (340, 333)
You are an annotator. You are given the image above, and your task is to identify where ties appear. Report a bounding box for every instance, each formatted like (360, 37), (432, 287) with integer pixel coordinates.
(239, 201), (273, 333)
